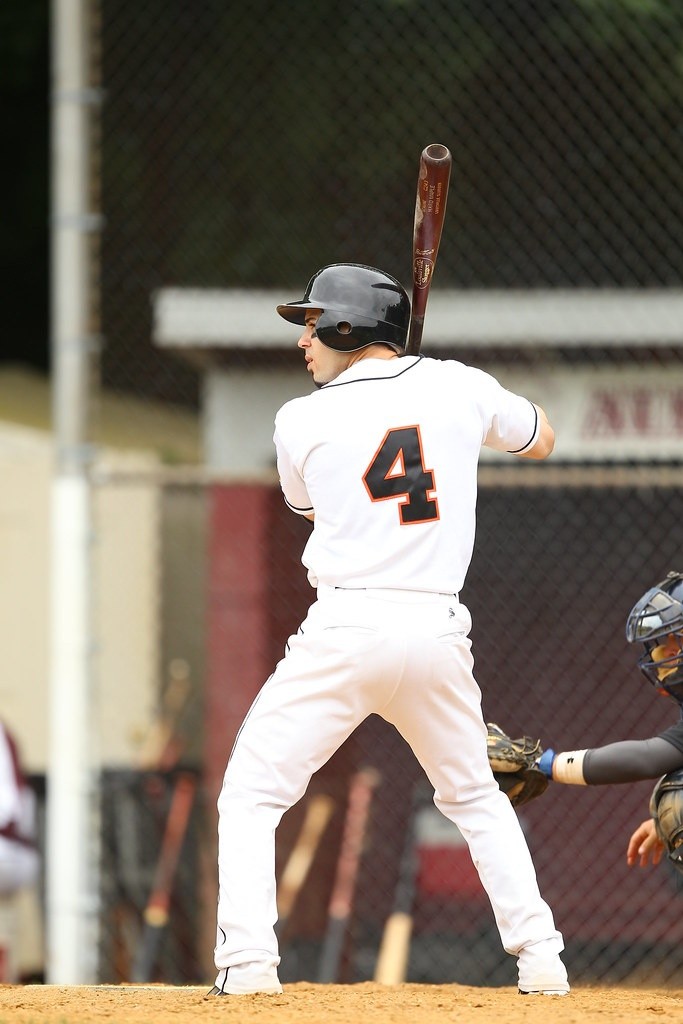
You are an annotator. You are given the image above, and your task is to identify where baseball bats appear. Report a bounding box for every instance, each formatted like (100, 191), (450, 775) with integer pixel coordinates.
(407, 142), (454, 357)
(120, 759), (197, 984)
(277, 766), (419, 986)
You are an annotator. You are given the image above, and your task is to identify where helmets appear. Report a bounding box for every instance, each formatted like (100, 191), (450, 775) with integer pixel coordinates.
(276, 261), (412, 355)
(625, 571), (682, 700)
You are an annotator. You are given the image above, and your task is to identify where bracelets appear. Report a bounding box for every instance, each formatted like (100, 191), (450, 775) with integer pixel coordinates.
(556, 748), (587, 786)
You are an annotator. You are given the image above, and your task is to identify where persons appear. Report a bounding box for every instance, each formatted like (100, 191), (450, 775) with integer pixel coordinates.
(208, 263), (575, 999)
(484, 569), (683, 870)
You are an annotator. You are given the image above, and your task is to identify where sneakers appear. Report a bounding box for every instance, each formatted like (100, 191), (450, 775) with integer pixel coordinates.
(515, 958), (571, 997)
(207, 963), (285, 997)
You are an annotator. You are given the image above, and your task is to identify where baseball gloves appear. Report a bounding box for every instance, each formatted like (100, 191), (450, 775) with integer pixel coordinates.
(485, 722), (551, 805)
